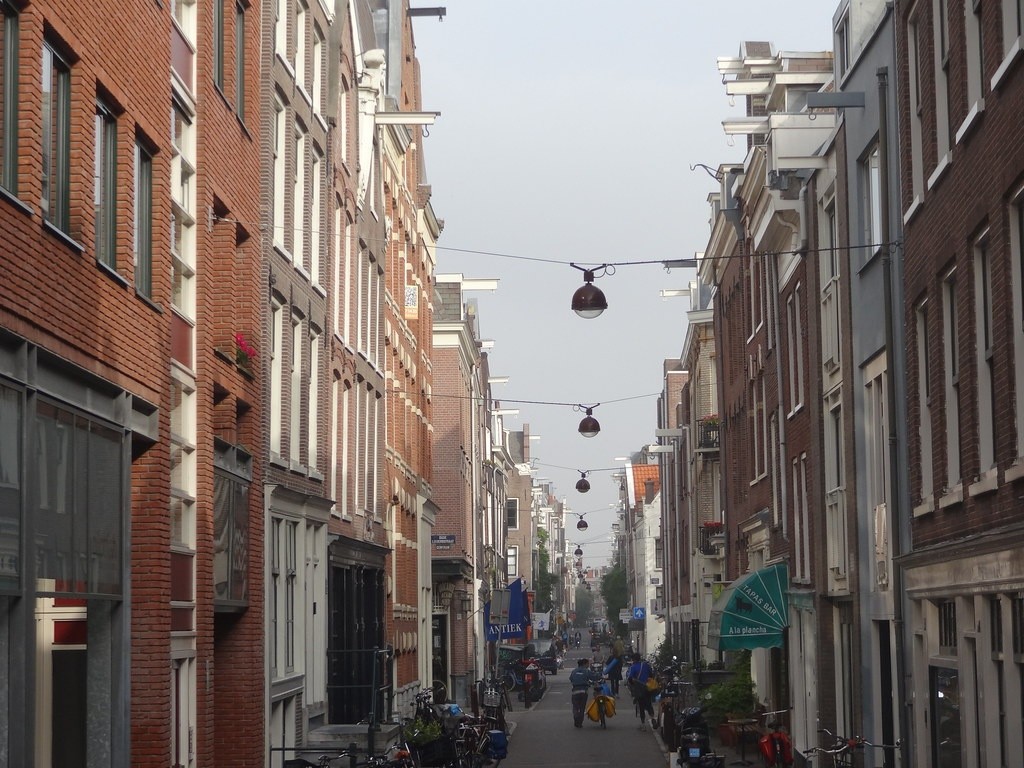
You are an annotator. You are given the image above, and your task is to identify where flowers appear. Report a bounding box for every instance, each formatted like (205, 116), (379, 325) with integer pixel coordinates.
(400, 716), (443, 742)
(704, 521), (720, 527)
(703, 414), (718, 426)
(235, 334), (256, 357)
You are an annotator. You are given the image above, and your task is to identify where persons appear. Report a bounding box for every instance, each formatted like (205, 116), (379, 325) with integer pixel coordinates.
(603, 640), (624, 698)
(626, 652), (658, 732)
(551, 630), (581, 652)
(589, 623), (607, 644)
(569, 659), (602, 728)
(653, 659), (675, 727)
(591, 644), (605, 672)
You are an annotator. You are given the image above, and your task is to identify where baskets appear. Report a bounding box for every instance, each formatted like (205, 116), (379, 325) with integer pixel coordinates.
(484, 694), (501, 706)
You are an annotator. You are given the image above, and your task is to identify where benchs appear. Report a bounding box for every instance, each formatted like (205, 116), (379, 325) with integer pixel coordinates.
(730, 703), (768, 742)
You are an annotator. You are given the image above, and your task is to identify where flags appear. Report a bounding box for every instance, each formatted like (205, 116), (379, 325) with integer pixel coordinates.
(521, 590), (531, 625)
(484, 578), (525, 640)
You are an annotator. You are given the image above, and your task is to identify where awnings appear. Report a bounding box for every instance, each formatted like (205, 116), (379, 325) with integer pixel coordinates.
(707, 562), (789, 651)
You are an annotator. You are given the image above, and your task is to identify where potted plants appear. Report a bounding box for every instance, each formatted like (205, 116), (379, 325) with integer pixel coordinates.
(699, 676), (757, 748)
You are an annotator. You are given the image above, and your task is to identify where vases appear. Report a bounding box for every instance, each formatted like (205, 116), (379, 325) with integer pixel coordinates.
(405, 739), (441, 767)
(704, 426), (718, 432)
(233, 350), (248, 366)
(702, 528), (718, 534)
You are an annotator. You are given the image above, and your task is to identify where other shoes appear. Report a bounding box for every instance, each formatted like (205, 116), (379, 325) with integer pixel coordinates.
(652, 719), (658, 729)
(640, 723), (646, 731)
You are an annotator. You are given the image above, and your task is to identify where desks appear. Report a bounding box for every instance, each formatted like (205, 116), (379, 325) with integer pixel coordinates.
(724, 717), (755, 765)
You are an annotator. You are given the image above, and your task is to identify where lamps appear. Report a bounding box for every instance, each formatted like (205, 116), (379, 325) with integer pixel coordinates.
(567, 262), (612, 319)
(576, 469), (590, 492)
(576, 514), (588, 531)
(579, 403), (601, 437)
(458, 593), (473, 614)
(575, 544), (583, 557)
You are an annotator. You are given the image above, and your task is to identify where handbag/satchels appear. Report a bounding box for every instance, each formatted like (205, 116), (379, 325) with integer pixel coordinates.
(646, 677), (660, 693)
(587, 695), (615, 721)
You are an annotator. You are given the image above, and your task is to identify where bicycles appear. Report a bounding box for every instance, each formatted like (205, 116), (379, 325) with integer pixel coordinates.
(588, 618), (901, 768)
(281, 632), (582, 768)
(586, 676), (607, 729)
